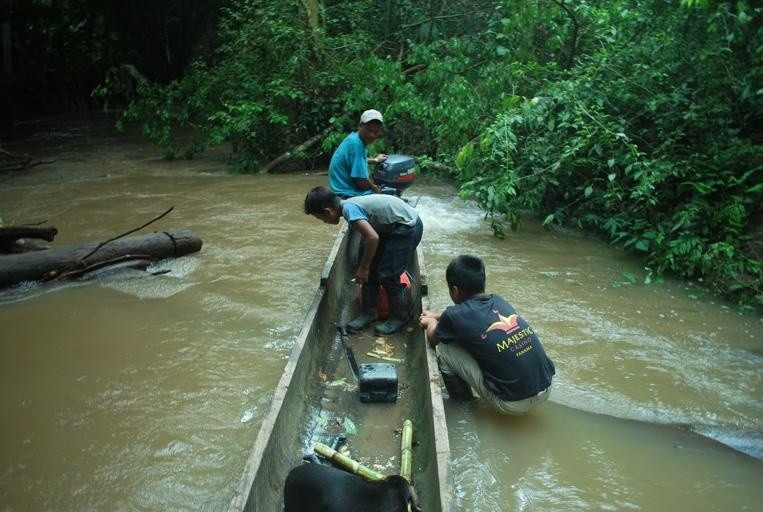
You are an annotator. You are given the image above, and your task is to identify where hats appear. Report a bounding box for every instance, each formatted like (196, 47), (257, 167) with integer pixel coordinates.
(358, 108), (384, 125)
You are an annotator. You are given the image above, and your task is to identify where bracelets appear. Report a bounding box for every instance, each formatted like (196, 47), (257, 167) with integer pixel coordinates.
(375, 158), (378, 163)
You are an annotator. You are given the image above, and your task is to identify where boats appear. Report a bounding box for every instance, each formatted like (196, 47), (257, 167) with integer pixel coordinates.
(227, 154), (458, 512)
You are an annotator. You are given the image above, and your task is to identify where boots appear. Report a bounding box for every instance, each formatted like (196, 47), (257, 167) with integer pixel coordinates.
(343, 281), (379, 334)
(373, 281), (412, 336)
(438, 370), (475, 404)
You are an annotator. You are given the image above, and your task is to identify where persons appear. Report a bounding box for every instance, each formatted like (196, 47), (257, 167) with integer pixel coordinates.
(304, 186), (423, 337)
(420, 254), (557, 415)
(327, 108), (388, 200)
